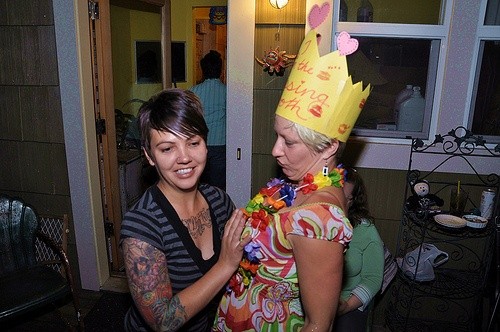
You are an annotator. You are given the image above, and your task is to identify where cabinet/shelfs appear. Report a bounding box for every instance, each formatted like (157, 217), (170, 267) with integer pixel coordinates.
(382, 126), (500, 332)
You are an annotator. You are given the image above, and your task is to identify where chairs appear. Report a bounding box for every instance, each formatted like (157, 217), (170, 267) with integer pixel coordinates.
(0, 194), (88, 332)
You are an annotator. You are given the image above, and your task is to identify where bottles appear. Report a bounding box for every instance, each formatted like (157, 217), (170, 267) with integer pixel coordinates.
(395, 84), (426, 132)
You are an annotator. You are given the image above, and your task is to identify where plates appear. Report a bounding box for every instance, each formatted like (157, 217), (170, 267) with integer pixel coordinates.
(434, 214), (487, 229)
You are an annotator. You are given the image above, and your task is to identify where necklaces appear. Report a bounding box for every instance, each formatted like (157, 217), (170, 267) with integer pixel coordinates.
(228, 164), (348, 293)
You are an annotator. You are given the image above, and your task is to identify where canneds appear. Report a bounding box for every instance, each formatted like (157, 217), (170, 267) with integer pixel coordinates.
(479, 189), (496, 220)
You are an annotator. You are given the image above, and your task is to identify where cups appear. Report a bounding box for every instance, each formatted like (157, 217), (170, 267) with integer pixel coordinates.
(449, 189), (470, 217)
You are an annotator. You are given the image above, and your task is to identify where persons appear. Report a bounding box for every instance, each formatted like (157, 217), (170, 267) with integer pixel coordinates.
(191, 50), (226, 192)
(120, 89), (253, 332)
(213, 115), (354, 332)
(332, 170), (385, 332)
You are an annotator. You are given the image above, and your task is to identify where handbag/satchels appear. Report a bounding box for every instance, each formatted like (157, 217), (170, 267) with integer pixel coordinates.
(396, 244), (448, 282)
